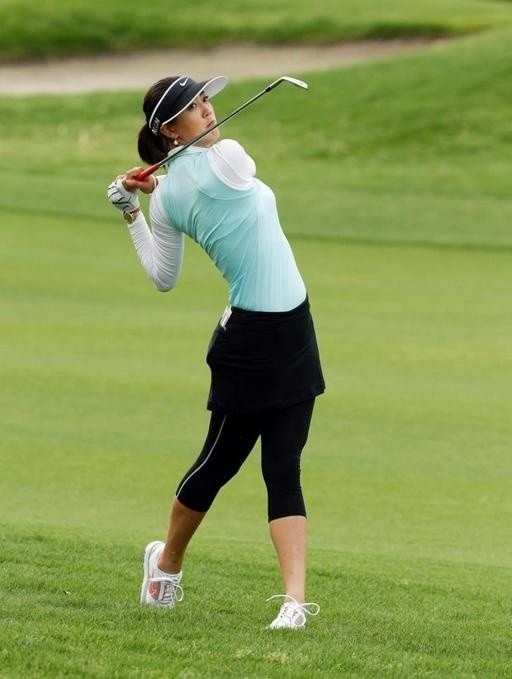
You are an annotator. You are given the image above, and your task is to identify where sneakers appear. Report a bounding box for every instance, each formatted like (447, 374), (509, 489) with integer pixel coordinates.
(140, 539), (185, 610)
(264, 599), (309, 633)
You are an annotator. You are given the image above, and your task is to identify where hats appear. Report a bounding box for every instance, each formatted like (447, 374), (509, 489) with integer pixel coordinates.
(146, 75), (228, 136)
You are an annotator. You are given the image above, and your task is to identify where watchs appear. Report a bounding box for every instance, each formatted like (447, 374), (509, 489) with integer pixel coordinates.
(123, 212), (139, 224)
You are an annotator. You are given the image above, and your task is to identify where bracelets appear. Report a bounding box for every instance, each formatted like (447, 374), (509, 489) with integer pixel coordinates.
(149, 173), (157, 195)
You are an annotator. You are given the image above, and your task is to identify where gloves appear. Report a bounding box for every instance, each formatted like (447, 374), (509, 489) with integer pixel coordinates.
(106, 174), (141, 225)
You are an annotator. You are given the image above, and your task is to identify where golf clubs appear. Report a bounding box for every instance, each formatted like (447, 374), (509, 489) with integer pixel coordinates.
(134, 75), (309, 182)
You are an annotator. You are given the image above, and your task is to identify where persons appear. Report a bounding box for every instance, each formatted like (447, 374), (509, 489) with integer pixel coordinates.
(107, 75), (324, 631)
